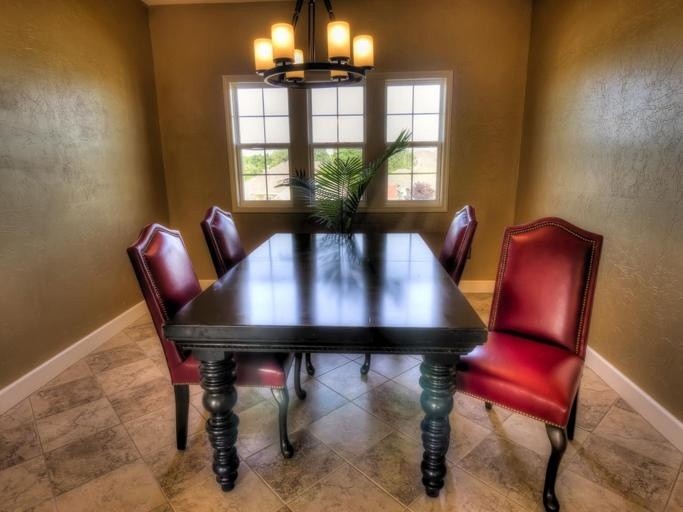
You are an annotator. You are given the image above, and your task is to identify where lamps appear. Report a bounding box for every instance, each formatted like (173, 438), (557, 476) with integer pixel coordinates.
(250, 1), (374, 90)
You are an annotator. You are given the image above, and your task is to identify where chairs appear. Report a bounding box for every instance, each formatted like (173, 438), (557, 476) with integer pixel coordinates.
(198, 206), (314, 376)
(360, 204), (479, 376)
(452, 216), (604, 512)
(125, 222), (305, 460)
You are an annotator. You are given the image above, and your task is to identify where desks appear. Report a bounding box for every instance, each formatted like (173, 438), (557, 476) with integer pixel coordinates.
(161, 233), (489, 497)
